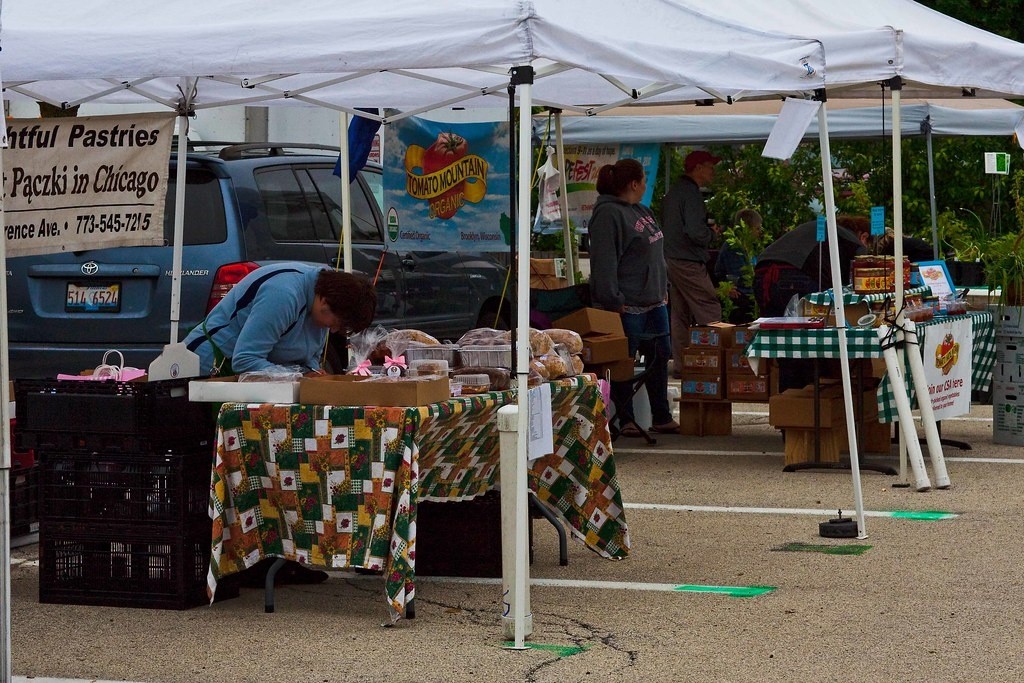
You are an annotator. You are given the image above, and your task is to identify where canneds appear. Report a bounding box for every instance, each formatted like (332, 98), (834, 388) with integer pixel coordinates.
(871, 293), (940, 321)
(851, 254), (910, 295)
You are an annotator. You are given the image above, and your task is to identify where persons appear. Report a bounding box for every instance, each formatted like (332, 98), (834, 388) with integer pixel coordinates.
(753, 217), (874, 395)
(181, 262), (375, 374)
(719, 209), (760, 321)
(659, 151), (723, 376)
(588, 158), (679, 436)
(877, 227), (933, 261)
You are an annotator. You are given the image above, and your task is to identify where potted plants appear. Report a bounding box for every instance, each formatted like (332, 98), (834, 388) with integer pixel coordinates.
(978, 232), (1024, 328)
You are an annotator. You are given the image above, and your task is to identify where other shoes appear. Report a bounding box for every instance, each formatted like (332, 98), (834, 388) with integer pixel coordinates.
(673, 370), (681, 379)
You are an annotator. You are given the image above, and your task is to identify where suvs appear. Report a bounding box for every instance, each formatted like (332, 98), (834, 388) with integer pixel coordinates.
(3, 139), (514, 393)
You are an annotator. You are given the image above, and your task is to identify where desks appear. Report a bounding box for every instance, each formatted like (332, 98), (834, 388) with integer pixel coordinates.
(743, 310), (998, 488)
(207, 373), (631, 628)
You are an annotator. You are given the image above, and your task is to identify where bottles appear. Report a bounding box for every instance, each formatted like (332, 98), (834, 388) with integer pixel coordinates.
(872, 293), (922, 327)
(852, 254), (910, 292)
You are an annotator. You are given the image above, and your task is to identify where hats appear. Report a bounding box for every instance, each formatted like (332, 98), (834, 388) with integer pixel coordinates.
(684, 151), (721, 171)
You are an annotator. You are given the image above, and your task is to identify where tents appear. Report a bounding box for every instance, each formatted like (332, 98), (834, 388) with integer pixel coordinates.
(1, 1), (1023, 651)
(537, 99), (1024, 490)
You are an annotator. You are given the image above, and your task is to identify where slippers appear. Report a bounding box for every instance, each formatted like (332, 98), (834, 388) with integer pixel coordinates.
(649, 424), (681, 435)
(620, 427), (642, 438)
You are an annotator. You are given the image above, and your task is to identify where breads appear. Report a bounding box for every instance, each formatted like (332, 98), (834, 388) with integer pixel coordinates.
(367, 325), (584, 387)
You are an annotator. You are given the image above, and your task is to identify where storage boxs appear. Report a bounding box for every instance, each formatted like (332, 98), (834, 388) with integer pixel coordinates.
(39, 524), (240, 611)
(726, 349), (768, 375)
(997, 337), (1024, 364)
(726, 375), (770, 400)
(552, 308), (634, 381)
(14, 378), (209, 452)
(688, 321), (736, 348)
(681, 348), (725, 374)
(770, 376), (883, 427)
(458, 345), (518, 367)
(732, 322), (753, 348)
(404, 343), (456, 368)
(993, 381), (1024, 446)
(993, 363), (1024, 384)
(681, 375), (725, 400)
(802, 300), (871, 327)
(299, 375), (449, 407)
(188, 376), (300, 404)
(986, 303), (1024, 337)
(33, 448), (213, 531)
